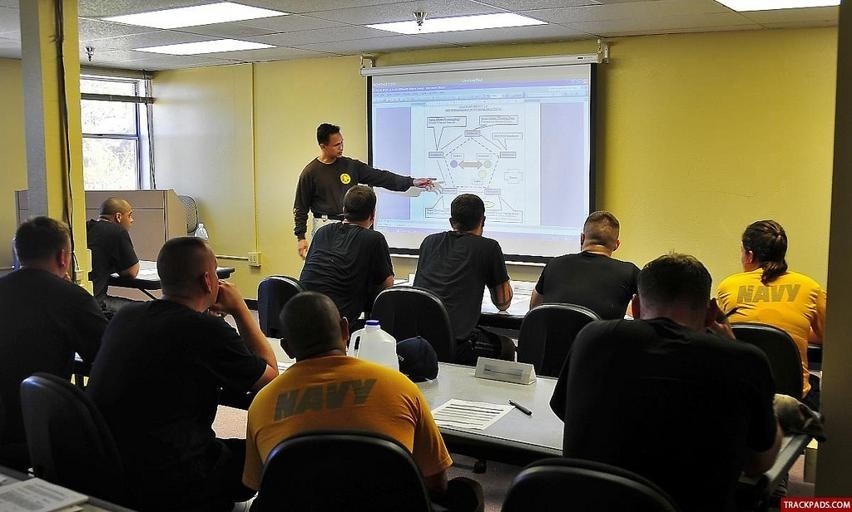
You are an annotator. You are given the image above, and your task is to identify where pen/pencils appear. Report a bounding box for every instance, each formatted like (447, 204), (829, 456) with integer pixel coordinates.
(509, 400), (532, 415)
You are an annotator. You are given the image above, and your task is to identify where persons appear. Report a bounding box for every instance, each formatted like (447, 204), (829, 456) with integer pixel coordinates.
(0, 217), (114, 471)
(242, 292), (453, 511)
(412, 195), (519, 363)
(547, 253), (785, 512)
(86, 196), (143, 323)
(294, 121), (437, 263)
(81, 232), (281, 512)
(711, 217), (827, 412)
(294, 182), (396, 341)
(527, 209), (645, 329)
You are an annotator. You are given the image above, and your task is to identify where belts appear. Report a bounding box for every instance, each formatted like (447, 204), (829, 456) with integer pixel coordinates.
(312, 212), (346, 221)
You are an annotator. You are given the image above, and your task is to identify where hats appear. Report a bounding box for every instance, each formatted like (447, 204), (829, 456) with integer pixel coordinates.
(396, 335), (439, 380)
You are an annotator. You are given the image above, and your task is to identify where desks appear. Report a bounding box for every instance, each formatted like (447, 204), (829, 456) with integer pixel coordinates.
(89, 259), (236, 300)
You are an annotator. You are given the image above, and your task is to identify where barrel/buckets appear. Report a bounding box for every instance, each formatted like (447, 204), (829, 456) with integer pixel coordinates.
(347, 321), (400, 372)
